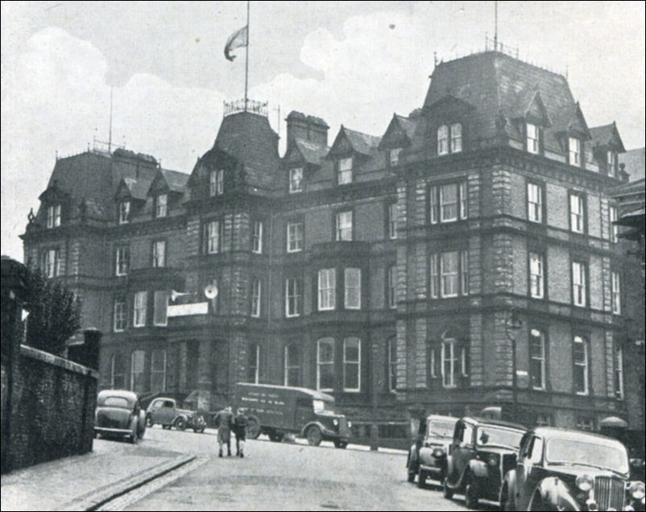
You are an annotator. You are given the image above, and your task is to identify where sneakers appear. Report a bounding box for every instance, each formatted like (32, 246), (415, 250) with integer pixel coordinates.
(219, 451), (244, 458)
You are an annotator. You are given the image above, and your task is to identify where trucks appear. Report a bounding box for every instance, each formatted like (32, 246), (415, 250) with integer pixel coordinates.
(235, 382), (351, 447)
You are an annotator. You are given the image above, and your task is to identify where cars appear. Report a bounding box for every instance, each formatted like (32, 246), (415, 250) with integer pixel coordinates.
(146, 398), (207, 432)
(498, 427), (646, 511)
(94, 389), (147, 445)
(445, 416), (527, 509)
(406, 415), (461, 489)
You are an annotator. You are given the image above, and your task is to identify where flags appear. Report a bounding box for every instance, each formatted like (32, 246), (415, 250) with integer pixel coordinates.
(223, 27), (250, 61)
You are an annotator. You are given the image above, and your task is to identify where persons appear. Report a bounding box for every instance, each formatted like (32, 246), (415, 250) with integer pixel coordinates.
(214, 405), (235, 457)
(230, 407), (249, 458)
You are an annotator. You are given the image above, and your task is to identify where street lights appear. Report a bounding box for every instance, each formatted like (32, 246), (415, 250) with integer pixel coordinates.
(505, 308), (525, 422)
